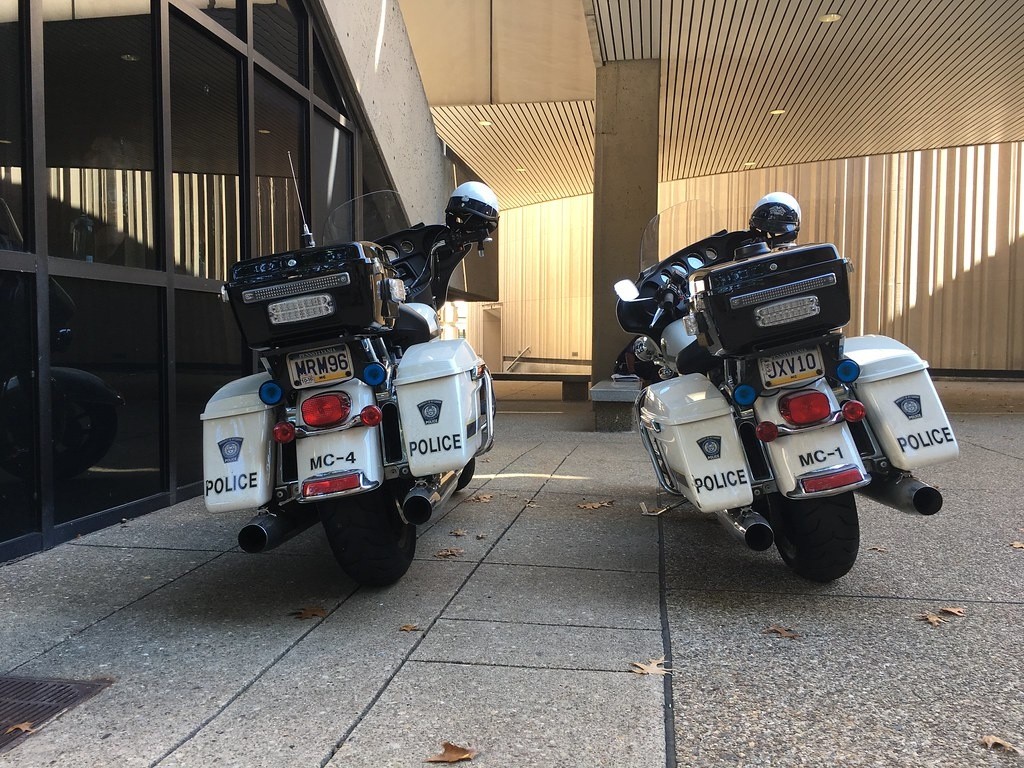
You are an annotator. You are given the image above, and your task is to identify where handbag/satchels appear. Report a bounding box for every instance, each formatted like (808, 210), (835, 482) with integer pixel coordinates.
(615, 351), (626, 375)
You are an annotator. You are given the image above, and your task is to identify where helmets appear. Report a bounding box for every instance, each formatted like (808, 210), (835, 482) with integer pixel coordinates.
(748, 191), (801, 239)
(445, 182), (498, 230)
(398, 299), (439, 345)
(661, 319), (697, 375)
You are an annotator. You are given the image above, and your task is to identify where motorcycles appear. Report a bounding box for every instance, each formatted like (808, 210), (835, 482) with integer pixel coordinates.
(613, 197), (962, 581)
(200, 189), (494, 590)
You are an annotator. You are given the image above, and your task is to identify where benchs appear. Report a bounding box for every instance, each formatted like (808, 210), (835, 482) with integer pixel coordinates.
(588, 380), (642, 431)
(492, 372), (592, 402)
